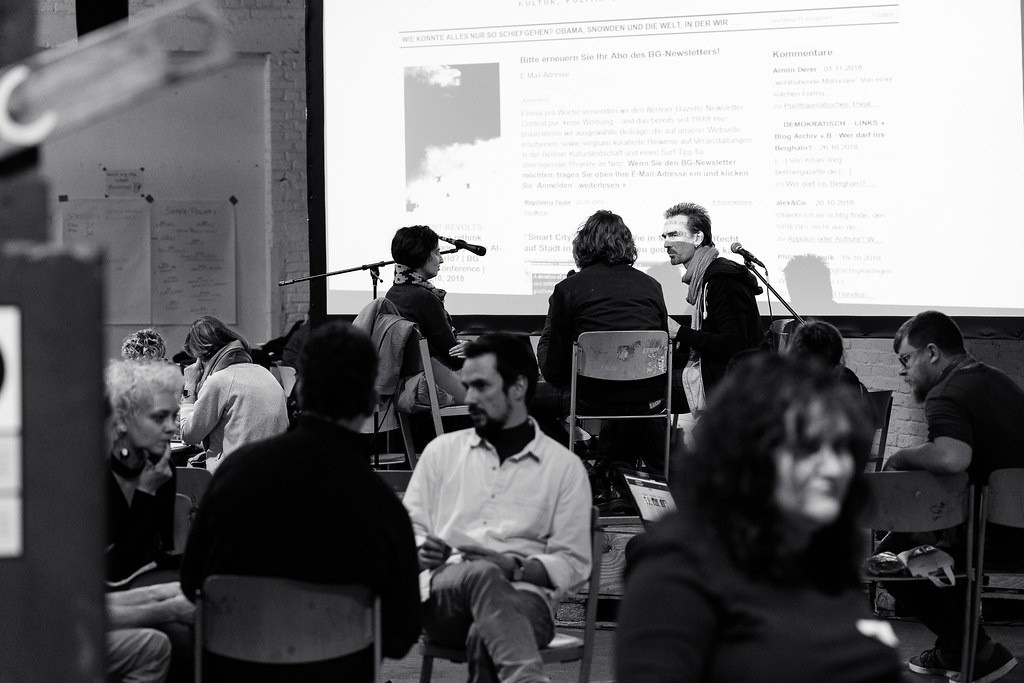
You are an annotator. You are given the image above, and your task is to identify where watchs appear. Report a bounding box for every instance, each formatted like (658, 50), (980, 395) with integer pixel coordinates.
(182, 390), (195, 398)
(510, 557), (524, 581)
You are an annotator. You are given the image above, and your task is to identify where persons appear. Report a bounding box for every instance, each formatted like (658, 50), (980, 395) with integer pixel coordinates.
(612, 352), (903, 683)
(179, 321), (422, 683)
(872, 310), (1024, 683)
(662, 202), (763, 415)
(180, 316), (290, 475)
(104, 358), (196, 683)
(534, 211), (669, 513)
(402, 333), (593, 683)
(784, 320), (846, 374)
(383, 225), (470, 406)
(121, 329), (166, 363)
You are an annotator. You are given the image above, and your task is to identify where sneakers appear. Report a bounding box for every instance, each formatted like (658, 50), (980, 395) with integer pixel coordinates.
(909, 629), (989, 674)
(940, 641), (1017, 682)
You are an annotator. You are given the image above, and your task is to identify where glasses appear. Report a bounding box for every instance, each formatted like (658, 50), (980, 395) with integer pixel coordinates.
(900, 341), (936, 369)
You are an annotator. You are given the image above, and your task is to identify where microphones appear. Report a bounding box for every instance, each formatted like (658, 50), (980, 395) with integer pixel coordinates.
(438, 236), (486, 256)
(731, 242), (765, 268)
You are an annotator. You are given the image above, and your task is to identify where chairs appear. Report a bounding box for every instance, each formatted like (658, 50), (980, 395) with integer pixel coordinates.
(168, 315), (1024, 683)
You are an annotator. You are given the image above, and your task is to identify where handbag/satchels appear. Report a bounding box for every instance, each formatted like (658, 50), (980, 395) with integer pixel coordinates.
(897, 546), (955, 587)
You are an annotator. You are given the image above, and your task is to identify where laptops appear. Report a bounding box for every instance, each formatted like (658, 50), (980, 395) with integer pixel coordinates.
(619, 467), (678, 531)
